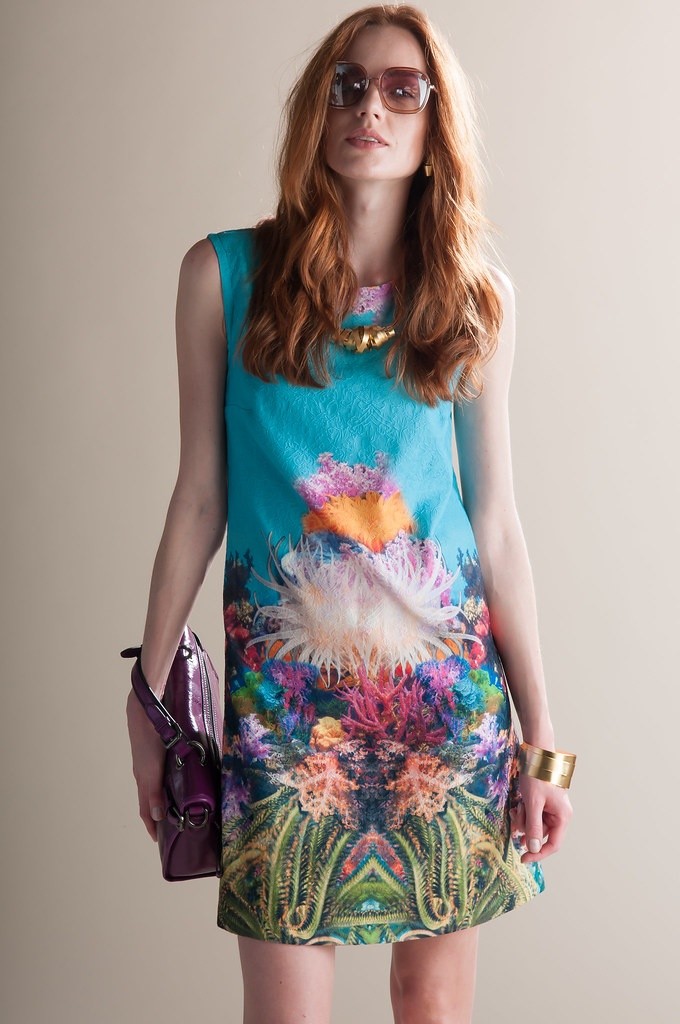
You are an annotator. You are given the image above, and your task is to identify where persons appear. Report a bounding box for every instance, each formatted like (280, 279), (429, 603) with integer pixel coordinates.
(125, 5), (572, 1024)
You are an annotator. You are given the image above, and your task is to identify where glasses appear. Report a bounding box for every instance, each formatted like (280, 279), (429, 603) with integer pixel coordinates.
(326, 59), (440, 113)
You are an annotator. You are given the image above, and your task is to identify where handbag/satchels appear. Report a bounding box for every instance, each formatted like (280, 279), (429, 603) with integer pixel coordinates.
(121, 626), (225, 883)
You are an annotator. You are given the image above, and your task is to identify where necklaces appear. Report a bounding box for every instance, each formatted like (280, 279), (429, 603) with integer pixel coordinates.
(329, 324), (395, 353)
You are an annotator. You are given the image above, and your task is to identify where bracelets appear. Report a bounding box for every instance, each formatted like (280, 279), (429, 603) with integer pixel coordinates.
(519, 741), (576, 789)
